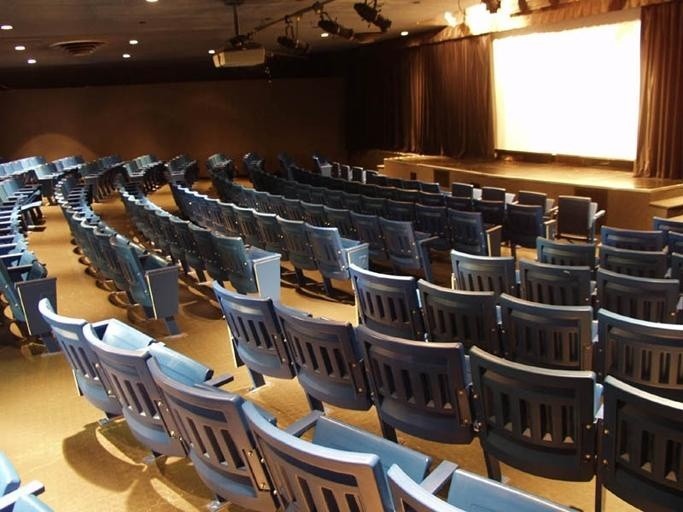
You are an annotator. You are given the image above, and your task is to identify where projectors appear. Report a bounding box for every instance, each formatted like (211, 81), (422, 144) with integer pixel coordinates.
(213, 46), (265, 67)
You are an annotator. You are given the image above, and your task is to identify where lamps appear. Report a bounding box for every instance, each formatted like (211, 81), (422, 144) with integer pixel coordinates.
(220, 0), (395, 55)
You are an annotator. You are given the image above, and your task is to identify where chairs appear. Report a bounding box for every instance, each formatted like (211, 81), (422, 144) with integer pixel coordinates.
(0, 149), (604, 355)
(537, 217), (682, 274)
(39, 249), (681, 510)
(2, 457), (51, 510)
